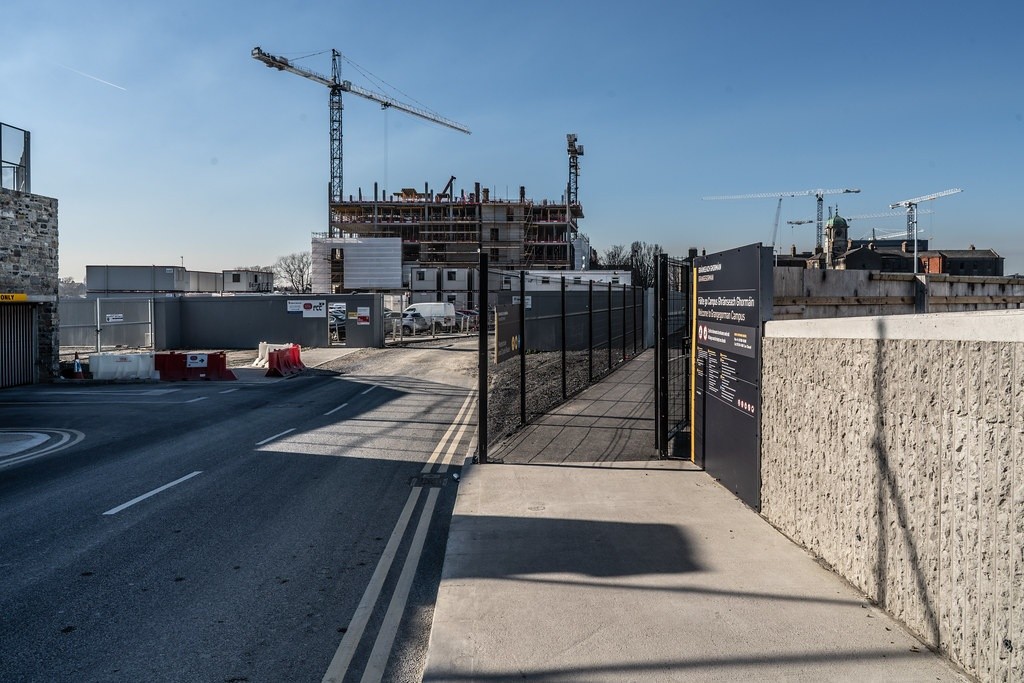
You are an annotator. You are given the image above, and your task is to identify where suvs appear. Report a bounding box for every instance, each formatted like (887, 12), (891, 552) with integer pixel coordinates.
(384, 311), (426, 336)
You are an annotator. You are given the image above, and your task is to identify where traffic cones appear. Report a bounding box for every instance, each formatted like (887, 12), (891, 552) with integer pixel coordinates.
(73, 351), (84, 379)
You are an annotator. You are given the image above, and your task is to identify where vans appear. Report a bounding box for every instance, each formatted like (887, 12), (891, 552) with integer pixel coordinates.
(403, 302), (455, 335)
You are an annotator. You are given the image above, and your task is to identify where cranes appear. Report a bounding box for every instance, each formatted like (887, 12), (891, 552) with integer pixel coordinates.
(699, 182), (965, 253)
(250, 41), (474, 258)
(562, 133), (585, 241)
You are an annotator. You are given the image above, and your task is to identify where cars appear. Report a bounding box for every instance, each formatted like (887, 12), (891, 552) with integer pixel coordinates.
(329, 310), (347, 340)
